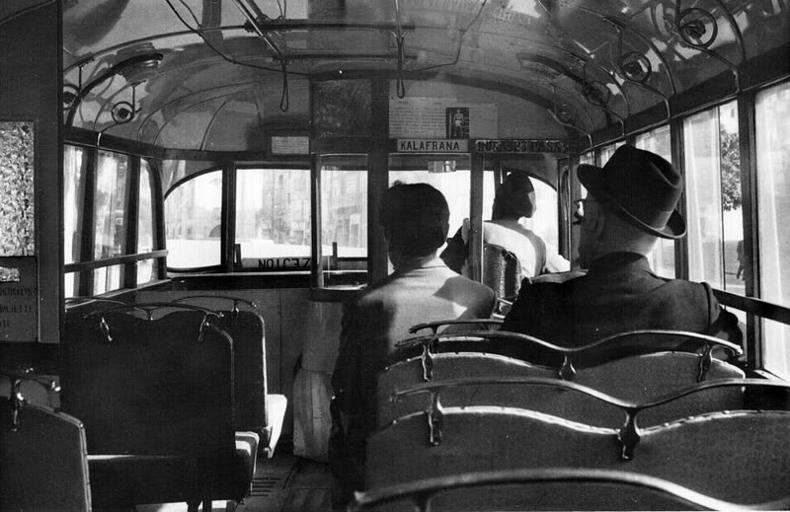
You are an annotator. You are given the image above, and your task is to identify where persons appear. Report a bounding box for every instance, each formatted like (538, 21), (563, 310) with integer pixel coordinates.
(736, 239), (745, 282)
(331, 179), (496, 459)
(438, 172), (547, 302)
(485, 143), (745, 366)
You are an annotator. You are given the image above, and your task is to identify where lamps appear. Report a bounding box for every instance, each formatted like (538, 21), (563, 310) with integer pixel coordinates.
(66, 42), (164, 131)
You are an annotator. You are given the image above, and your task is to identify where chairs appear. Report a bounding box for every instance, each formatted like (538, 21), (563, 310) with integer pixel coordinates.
(1, 243), (789, 511)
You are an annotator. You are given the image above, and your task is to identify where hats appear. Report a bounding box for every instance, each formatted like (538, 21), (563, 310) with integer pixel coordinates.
(578, 144), (680, 239)
(502, 172), (535, 217)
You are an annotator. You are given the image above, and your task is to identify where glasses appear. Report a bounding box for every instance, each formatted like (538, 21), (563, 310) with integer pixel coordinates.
(573, 197), (594, 222)
